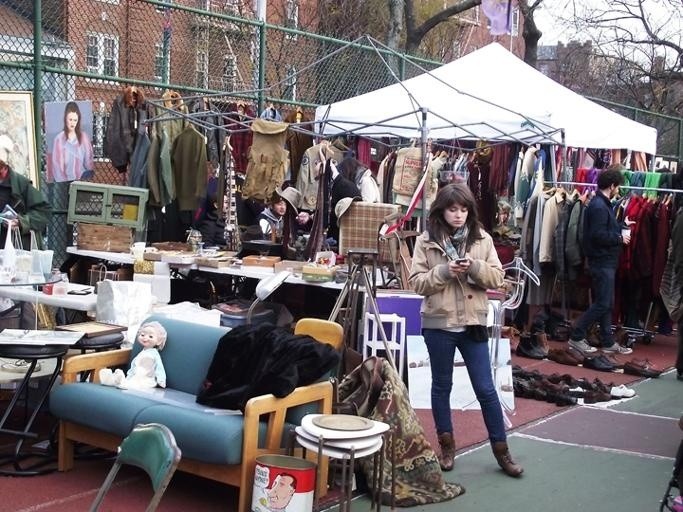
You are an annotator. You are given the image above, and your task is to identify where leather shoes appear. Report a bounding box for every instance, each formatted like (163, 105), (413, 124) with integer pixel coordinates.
(511, 364), (636, 408)
(623, 358), (664, 379)
(546, 345), (584, 366)
(582, 352), (626, 373)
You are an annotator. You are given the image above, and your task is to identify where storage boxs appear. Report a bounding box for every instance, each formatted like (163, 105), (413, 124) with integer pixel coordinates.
(209, 303), (275, 329)
(76, 224), (134, 253)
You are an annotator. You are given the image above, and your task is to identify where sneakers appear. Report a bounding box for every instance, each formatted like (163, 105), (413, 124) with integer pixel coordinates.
(600, 341), (633, 355)
(567, 337), (598, 354)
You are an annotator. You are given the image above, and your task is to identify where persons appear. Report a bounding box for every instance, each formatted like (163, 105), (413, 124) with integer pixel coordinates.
(53, 102), (93, 182)
(0, 159), (53, 292)
(407, 183), (525, 476)
(517, 305), (550, 359)
(567, 169), (637, 355)
(257, 192), (289, 243)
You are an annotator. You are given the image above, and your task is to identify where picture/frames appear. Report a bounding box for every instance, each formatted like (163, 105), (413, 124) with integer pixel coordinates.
(0, 91), (40, 192)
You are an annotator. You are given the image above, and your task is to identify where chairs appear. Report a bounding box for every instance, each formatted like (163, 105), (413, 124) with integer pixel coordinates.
(294, 318), (348, 351)
(87, 421), (184, 512)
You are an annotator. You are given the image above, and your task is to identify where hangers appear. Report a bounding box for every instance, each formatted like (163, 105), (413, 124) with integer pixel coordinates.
(425, 141), (494, 170)
(115, 79), (359, 157)
(523, 177), (682, 219)
(514, 140), (547, 183)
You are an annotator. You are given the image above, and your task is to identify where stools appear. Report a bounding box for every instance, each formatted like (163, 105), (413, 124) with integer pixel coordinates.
(58, 326), (126, 386)
(282, 410), (397, 511)
(0, 330), (67, 477)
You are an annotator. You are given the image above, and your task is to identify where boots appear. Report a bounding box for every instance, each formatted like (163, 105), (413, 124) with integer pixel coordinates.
(500, 325), (520, 353)
(489, 437), (524, 477)
(515, 330), (549, 360)
(435, 429), (455, 471)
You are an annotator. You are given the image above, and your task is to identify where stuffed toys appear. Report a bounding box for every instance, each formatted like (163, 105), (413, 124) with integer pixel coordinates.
(100, 321), (168, 391)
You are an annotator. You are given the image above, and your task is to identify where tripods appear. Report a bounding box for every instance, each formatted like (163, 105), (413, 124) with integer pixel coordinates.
(327, 247), (397, 378)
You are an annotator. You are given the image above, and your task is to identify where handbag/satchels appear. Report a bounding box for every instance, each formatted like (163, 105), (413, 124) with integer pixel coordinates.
(0, 219), (56, 305)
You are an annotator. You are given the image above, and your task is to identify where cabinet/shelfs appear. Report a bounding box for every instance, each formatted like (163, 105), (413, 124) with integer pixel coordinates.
(364, 310), (406, 381)
(356, 288), (497, 394)
(66, 181), (150, 231)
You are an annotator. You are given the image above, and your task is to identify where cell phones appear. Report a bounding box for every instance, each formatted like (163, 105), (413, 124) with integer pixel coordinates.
(455, 259), (467, 265)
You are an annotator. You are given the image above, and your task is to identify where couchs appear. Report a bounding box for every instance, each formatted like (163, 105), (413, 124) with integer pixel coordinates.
(51, 314), (335, 511)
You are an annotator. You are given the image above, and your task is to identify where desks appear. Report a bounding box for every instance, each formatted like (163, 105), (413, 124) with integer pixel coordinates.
(0, 277), (98, 316)
(65, 242), (387, 315)
(0, 354), (64, 383)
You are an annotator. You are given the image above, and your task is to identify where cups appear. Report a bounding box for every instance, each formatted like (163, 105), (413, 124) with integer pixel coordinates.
(132, 241), (146, 261)
(620, 228), (632, 237)
(1, 244), (55, 281)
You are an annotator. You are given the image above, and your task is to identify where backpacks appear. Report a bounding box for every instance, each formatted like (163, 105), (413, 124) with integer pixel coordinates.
(241, 118), (292, 202)
